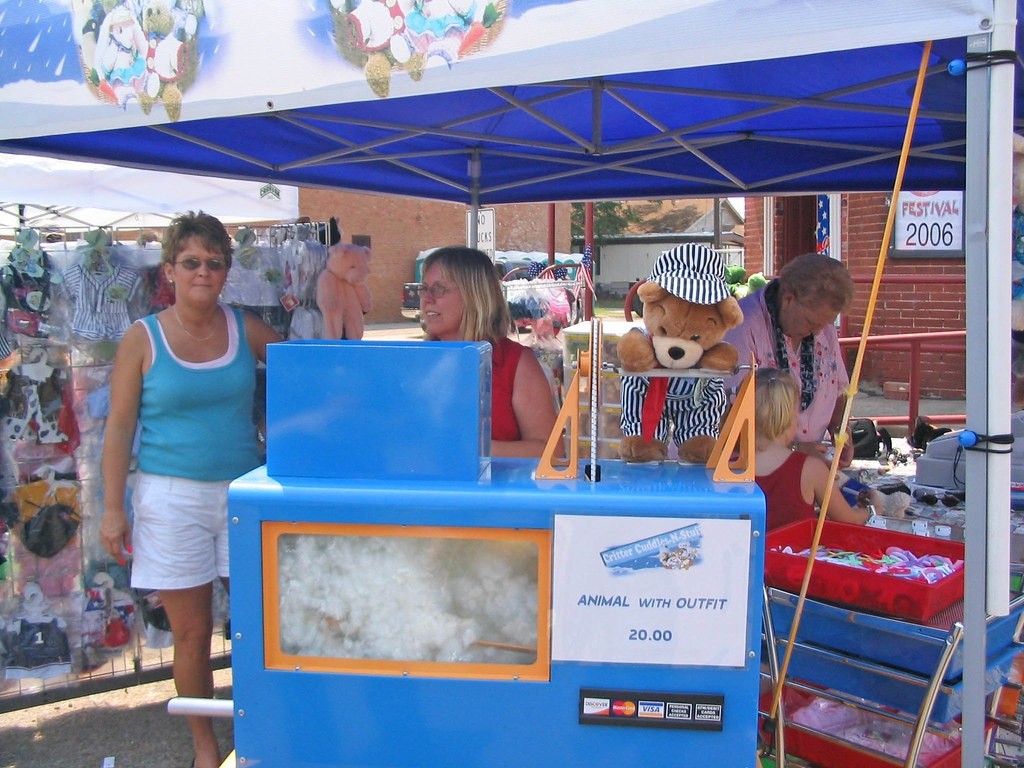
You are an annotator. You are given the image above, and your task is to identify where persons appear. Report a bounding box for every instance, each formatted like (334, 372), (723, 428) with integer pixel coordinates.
(100, 209), (286, 768)
(419, 243), (566, 463)
(729, 368), (882, 536)
(721, 254), (853, 473)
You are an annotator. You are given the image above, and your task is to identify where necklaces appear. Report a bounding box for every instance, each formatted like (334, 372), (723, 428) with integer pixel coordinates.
(173, 307), (223, 342)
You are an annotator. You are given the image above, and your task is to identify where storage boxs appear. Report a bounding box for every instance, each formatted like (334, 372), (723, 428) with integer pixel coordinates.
(753, 514), (1024, 768)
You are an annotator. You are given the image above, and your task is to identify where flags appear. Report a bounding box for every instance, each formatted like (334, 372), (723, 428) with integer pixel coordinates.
(579, 243), (598, 302)
(528, 261), (568, 279)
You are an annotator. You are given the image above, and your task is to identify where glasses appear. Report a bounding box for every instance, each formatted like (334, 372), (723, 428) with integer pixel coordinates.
(415, 283), (452, 297)
(174, 258), (228, 271)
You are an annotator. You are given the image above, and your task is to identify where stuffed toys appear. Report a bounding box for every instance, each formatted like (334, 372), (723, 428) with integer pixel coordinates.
(616, 244), (746, 464)
(315, 242), (373, 341)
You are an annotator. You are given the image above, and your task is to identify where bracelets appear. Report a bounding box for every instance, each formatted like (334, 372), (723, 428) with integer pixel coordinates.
(792, 440), (799, 452)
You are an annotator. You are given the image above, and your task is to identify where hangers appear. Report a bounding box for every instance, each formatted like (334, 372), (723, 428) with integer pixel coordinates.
(31, 466), (73, 488)
(5, 571), (151, 633)
(260, 220), (327, 251)
(11, 346), (71, 381)
(63, 245), (139, 281)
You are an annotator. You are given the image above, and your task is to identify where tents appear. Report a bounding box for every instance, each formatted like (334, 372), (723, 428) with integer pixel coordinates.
(0, 0), (1024, 768)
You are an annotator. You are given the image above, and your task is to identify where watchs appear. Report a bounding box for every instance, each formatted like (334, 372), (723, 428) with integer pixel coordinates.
(864, 505), (876, 519)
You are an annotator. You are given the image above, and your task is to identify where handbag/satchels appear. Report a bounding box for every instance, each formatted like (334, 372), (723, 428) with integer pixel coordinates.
(7, 307), (40, 336)
(20, 491), (83, 558)
